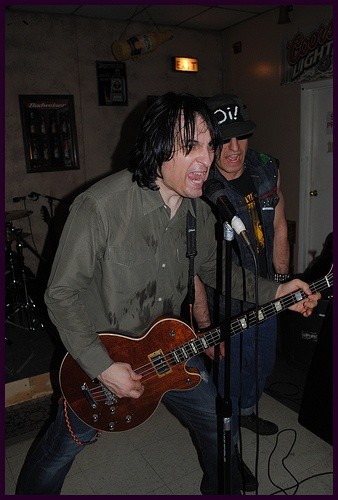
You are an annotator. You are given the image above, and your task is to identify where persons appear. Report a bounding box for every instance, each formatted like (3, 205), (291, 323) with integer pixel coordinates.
(190, 104), (289, 492)
(15, 91), (321, 496)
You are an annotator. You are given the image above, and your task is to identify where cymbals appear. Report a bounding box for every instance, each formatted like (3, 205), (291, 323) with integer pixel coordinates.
(5, 208), (34, 222)
(5, 232), (32, 241)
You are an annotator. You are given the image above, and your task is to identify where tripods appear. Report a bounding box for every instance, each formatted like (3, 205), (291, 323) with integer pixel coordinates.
(6, 222), (45, 330)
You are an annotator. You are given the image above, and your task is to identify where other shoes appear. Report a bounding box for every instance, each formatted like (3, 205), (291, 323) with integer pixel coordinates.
(227, 443), (259, 492)
(200, 475), (242, 495)
(239, 412), (279, 435)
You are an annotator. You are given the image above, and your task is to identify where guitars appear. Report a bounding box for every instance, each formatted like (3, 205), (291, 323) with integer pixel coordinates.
(59, 264), (335, 432)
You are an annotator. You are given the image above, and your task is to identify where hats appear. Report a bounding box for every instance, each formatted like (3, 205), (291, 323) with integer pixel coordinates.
(205, 94), (256, 140)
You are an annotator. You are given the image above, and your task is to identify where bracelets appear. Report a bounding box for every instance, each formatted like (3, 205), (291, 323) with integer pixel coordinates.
(274, 273), (289, 282)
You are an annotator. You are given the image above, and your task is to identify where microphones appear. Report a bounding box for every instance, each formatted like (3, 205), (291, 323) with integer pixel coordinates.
(202, 178), (250, 246)
